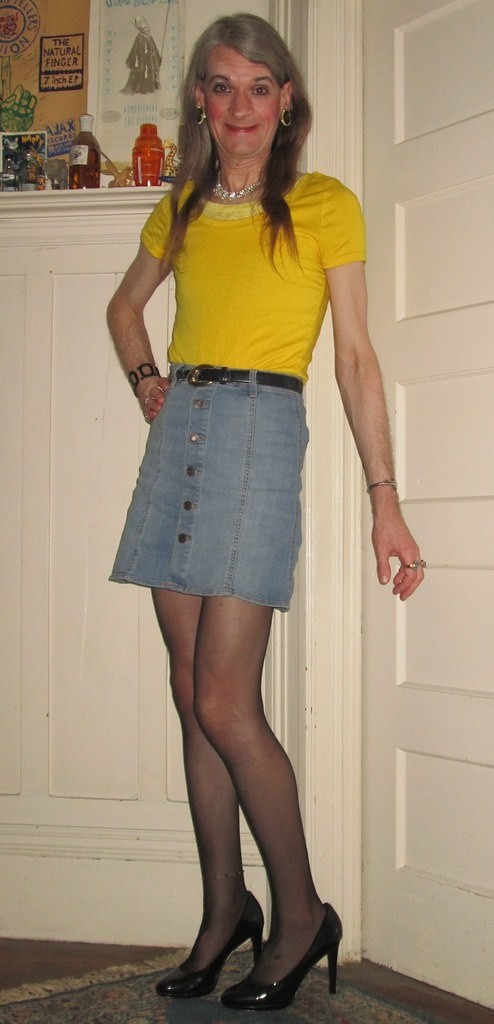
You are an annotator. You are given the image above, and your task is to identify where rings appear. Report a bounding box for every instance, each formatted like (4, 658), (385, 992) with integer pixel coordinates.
(420, 560), (426, 567)
(144, 408), (150, 418)
(144, 397), (149, 403)
(408, 560), (419, 570)
(157, 386), (168, 392)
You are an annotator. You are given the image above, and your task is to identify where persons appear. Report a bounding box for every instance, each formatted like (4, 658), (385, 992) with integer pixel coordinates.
(106, 13), (425, 1009)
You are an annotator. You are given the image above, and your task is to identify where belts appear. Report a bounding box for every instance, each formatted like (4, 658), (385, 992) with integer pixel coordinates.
(175, 364), (304, 395)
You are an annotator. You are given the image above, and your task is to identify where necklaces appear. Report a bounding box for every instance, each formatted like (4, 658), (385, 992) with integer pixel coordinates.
(212, 179), (263, 200)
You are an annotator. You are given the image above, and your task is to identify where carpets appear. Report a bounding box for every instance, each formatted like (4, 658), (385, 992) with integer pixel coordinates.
(0, 938), (434, 1024)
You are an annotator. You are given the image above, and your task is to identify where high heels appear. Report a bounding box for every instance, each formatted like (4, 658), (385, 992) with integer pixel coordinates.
(220, 902), (342, 1012)
(155, 889), (265, 998)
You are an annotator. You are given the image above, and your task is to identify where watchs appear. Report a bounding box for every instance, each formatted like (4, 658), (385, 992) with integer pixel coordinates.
(368, 479), (397, 492)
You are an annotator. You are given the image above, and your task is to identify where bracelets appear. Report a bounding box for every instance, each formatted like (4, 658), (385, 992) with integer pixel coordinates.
(128, 364), (161, 398)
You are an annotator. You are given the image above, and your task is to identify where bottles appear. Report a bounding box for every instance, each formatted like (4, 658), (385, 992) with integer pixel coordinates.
(68, 113), (101, 189)
(2, 159), (16, 193)
(17, 153), (38, 191)
(132, 123), (165, 186)
